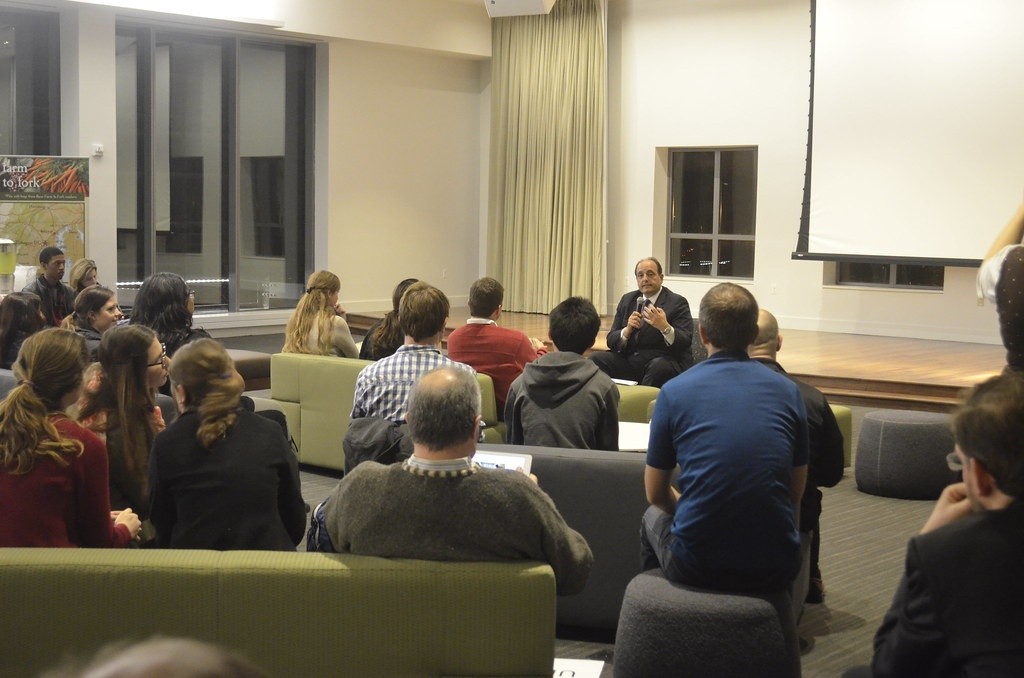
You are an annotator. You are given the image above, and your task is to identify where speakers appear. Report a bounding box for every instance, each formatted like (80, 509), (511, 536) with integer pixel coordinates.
(484, 0), (556, 19)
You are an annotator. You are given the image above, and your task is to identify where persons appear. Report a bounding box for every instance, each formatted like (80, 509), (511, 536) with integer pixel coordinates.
(60, 285), (121, 360)
(843, 374), (1024, 678)
(747, 309), (845, 605)
(350, 282), (477, 423)
(360, 277), (419, 360)
(0, 291), (46, 401)
(588, 257), (694, 390)
(504, 297), (620, 451)
(0, 328), (141, 549)
(81, 633), (261, 678)
(69, 258), (101, 297)
(21, 246), (76, 330)
(447, 278), (548, 425)
(307, 368), (595, 611)
(976, 204), (1024, 375)
(148, 338), (306, 552)
(284, 269), (358, 359)
(129, 272), (310, 513)
(72, 323), (171, 549)
(637, 283), (809, 653)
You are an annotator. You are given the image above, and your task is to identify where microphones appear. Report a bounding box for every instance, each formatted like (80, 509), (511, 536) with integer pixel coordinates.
(636, 296), (645, 314)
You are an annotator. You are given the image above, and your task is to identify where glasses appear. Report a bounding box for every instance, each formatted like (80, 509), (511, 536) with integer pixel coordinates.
(945, 450), (996, 472)
(189, 290), (195, 298)
(148, 343), (166, 368)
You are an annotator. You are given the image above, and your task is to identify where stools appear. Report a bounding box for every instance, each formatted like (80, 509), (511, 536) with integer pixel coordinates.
(227, 348), (959, 678)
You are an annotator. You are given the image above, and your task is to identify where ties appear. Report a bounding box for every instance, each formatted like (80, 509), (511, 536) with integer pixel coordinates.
(643, 299), (650, 311)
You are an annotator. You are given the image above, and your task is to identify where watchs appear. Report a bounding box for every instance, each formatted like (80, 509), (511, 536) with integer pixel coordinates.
(660, 324), (671, 334)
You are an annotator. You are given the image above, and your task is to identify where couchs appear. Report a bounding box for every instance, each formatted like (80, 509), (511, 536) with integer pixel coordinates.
(0, 547), (559, 678)
(349, 416), (814, 634)
(243, 350), (510, 471)
